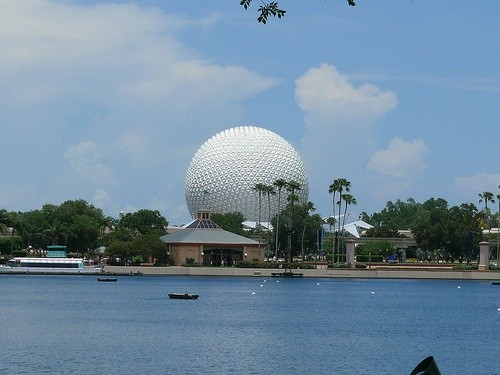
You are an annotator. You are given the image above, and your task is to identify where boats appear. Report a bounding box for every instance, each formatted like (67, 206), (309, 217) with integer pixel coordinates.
(491, 282), (500, 285)
(97, 277), (118, 282)
(168, 293), (199, 300)
